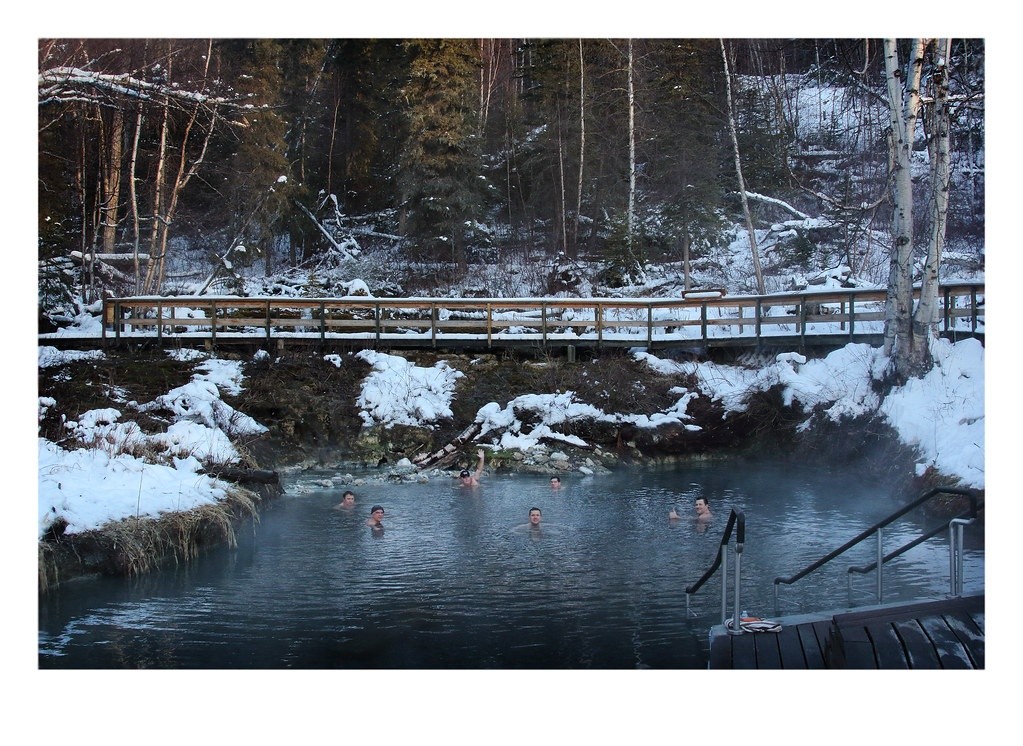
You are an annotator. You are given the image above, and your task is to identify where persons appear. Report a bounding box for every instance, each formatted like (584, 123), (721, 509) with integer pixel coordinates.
(340, 491), (354, 505)
(528, 507), (542, 525)
(670, 497), (712, 518)
(369, 505), (384, 524)
(460, 449), (484, 486)
(550, 476), (561, 487)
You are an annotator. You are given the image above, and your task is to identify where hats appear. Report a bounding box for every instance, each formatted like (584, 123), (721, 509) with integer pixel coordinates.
(370, 505), (384, 515)
(460, 471), (469, 478)
